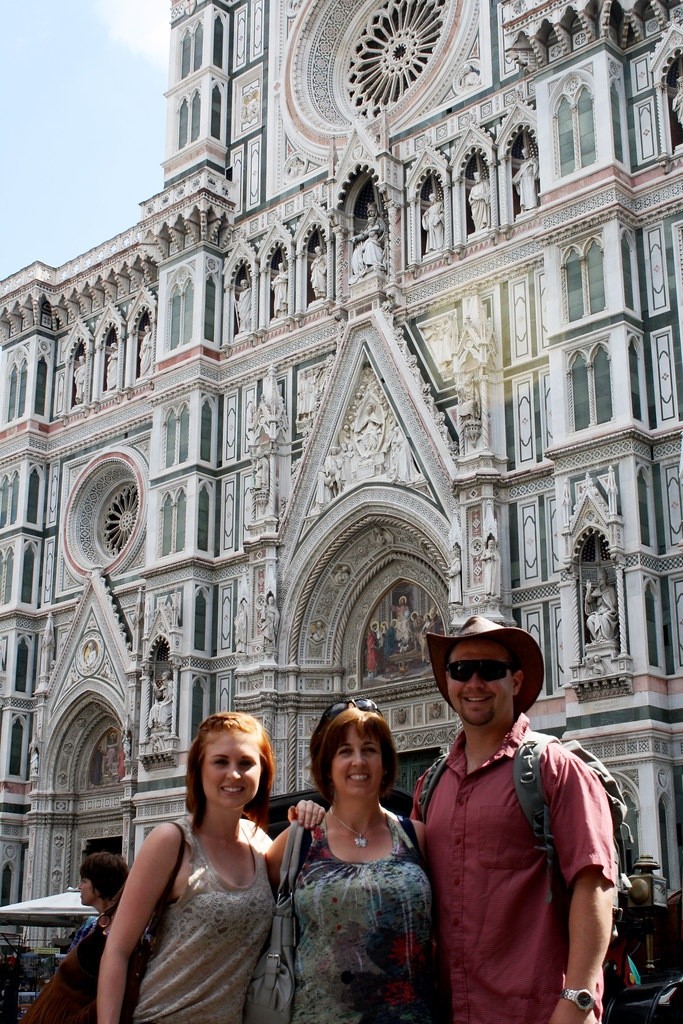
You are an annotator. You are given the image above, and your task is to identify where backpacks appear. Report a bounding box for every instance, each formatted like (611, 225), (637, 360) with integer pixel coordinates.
(415, 740), (633, 943)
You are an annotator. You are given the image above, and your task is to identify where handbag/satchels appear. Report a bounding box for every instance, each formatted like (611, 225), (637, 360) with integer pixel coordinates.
(18, 822), (185, 1024)
(245, 819), (304, 1024)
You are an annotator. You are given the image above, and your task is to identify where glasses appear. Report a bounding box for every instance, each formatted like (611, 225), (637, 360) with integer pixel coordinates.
(444, 658), (517, 682)
(316, 698), (385, 734)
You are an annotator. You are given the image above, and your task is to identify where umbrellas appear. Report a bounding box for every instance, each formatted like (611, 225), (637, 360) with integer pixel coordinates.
(0, 887), (101, 928)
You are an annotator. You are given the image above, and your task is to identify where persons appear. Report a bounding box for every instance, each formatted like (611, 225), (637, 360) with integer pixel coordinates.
(74, 356), (87, 404)
(310, 245), (328, 299)
(271, 263), (288, 318)
(0, 958), (40, 1024)
(234, 597), (280, 653)
(413, 617), (618, 1024)
(148, 672), (174, 729)
(69, 852), (151, 950)
(448, 540), (501, 605)
(263, 704), (438, 1024)
(603, 930), (641, 1001)
(672, 77), (683, 129)
(139, 325), (151, 377)
(584, 568), (618, 641)
(349, 148), (538, 285)
(235, 278), (251, 333)
(98, 712), (326, 1024)
(458, 373), (481, 419)
(254, 444), (270, 492)
(107, 342), (118, 390)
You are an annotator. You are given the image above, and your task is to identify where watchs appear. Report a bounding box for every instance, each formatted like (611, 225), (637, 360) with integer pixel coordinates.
(560, 988), (597, 1012)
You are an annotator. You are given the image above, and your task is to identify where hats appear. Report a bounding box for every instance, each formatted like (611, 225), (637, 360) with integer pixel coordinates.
(427, 615), (545, 714)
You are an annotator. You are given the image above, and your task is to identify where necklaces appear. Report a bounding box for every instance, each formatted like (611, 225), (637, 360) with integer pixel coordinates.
(330, 807), (385, 848)
